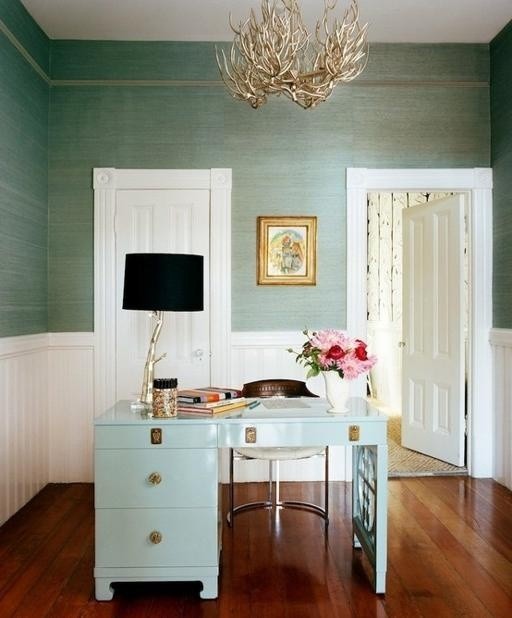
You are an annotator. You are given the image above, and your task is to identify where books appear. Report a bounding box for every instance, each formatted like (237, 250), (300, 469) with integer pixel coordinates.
(173, 386), (251, 414)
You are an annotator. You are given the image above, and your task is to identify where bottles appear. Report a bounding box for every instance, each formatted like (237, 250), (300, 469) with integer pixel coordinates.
(151, 376), (179, 418)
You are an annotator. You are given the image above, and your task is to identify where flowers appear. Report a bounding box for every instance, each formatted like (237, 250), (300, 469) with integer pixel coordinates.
(285, 324), (377, 380)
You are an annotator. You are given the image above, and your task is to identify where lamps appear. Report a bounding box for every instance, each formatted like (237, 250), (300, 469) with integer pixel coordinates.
(122, 252), (204, 410)
(213, 0), (370, 111)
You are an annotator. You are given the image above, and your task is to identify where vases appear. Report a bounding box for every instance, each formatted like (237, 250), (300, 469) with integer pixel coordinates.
(321, 371), (352, 414)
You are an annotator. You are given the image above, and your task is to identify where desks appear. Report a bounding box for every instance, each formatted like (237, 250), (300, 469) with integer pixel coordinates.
(92, 399), (390, 602)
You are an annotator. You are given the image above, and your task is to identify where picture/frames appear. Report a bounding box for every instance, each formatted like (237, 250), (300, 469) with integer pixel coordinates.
(255, 215), (317, 287)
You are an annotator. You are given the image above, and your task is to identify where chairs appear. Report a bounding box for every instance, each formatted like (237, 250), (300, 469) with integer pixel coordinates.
(225, 379), (329, 528)
